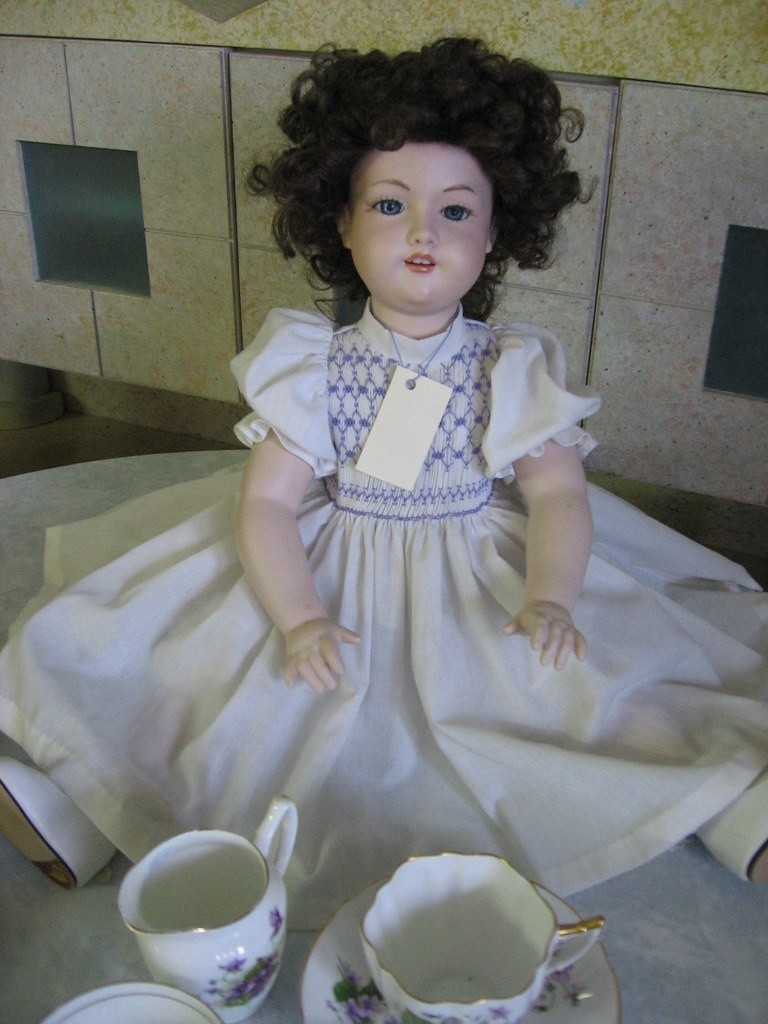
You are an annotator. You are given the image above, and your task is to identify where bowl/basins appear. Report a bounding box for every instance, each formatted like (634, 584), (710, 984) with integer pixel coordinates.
(37, 981), (224, 1022)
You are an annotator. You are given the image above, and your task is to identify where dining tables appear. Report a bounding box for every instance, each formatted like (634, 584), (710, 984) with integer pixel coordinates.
(1, 447), (767, 1024)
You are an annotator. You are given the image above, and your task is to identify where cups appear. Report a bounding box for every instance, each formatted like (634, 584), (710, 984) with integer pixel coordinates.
(356, 849), (605, 1024)
(115, 795), (301, 1023)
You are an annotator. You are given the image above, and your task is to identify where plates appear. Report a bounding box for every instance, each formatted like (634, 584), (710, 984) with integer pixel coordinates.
(299, 871), (622, 1024)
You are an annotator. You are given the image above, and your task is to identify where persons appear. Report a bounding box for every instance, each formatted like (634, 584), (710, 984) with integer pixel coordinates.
(0, 35), (767, 895)
